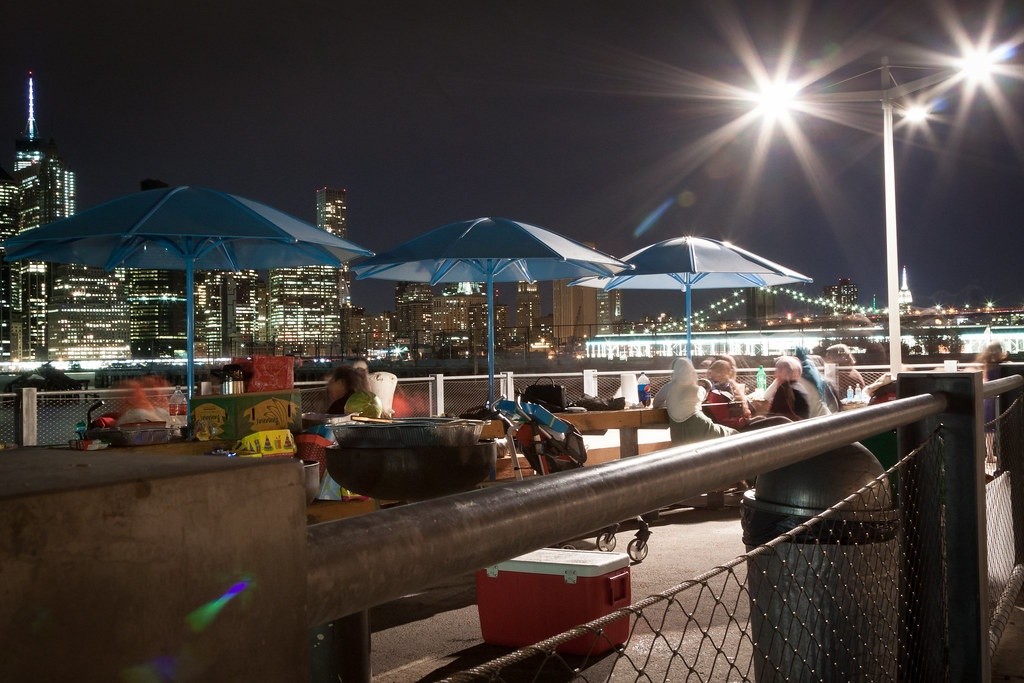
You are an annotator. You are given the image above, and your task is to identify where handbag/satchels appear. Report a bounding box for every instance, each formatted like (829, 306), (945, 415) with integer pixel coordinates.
(578, 394), (626, 410)
(523, 376), (567, 413)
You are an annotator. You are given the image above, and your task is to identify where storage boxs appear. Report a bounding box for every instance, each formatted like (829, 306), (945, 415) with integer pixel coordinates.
(476, 550), (631, 654)
(189, 355), (302, 440)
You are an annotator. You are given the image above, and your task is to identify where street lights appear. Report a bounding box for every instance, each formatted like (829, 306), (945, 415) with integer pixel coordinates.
(741, 36), (1023, 380)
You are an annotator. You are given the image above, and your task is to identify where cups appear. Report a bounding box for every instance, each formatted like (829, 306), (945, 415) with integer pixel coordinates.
(944, 359), (957, 372)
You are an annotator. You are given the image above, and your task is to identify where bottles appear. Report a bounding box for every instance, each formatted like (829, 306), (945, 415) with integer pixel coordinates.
(847, 386), (853, 399)
(638, 372), (651, 406)
(75, 421), (86, 441)
(757, 365), (766, 389)
(169, 386), (188, 436)
(855, 384), (861, 396)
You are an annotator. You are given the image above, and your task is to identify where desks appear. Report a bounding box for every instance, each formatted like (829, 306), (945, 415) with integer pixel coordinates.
(481, 400), (770, 459)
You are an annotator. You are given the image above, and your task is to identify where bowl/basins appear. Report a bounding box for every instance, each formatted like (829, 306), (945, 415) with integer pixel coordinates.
(324, 439), (498, 498)
(304, 460), (320, 505)
(87, 421), (176, 446)
(325, 418), (493, 446)
(220, 380), (245, 395)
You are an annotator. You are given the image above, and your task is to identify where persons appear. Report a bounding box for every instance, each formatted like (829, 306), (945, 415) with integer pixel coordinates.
(705, 355), (766, 429)
(975, 342), (1008, 463)
(653, 357), (740, 446)
(308, 365), (372, 442)
(826, 344), (865, 399)
(764, 349), (830, 419)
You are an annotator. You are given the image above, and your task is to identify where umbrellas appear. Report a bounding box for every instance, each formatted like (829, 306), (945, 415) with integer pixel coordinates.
(349, 215), (637, 404)
(568, 235), (814, 358)
(0, 183), (377, 426)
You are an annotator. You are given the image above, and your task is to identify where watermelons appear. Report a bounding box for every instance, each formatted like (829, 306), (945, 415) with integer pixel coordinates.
(344, 390), (382, 419)
